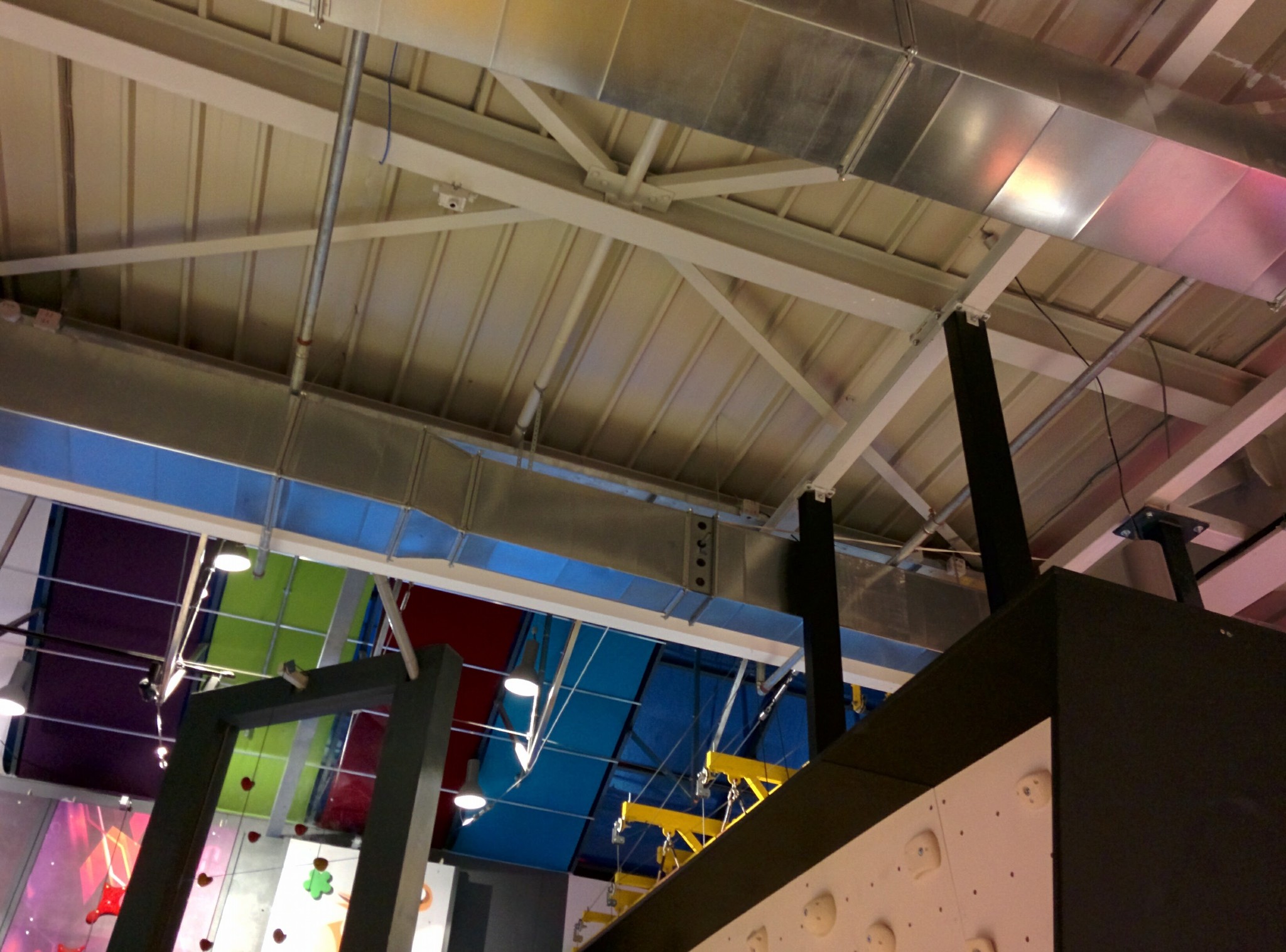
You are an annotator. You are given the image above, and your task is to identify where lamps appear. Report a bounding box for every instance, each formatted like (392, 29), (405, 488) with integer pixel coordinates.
(505, 627), (542, 697)
(212, 541), (251, 572)
(0, 636), (47, 715)
(138, 660), (163, 702)
(454, 754), (486, 811)
